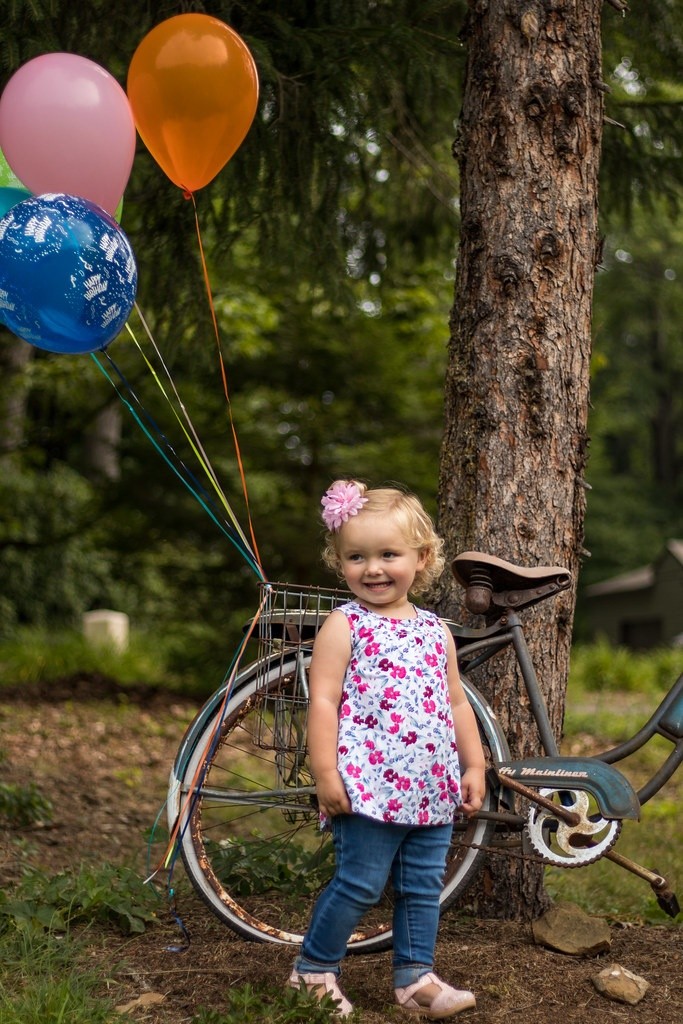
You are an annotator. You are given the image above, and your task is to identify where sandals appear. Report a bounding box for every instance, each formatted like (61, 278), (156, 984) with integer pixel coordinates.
(285, 969), (353, 1021)
(394, 972), (476, 1019)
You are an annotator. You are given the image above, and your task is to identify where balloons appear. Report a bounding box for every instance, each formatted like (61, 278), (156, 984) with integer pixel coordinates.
(0, 193), (137, 356)
(0, 52), (137, 227)
(126, 13), (259, 195)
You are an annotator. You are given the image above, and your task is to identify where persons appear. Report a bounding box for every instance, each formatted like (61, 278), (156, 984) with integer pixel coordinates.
(288, 479), (486, 1020)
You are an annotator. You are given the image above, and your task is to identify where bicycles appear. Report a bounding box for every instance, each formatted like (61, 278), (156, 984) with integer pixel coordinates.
(165, 538), (682, 956)
